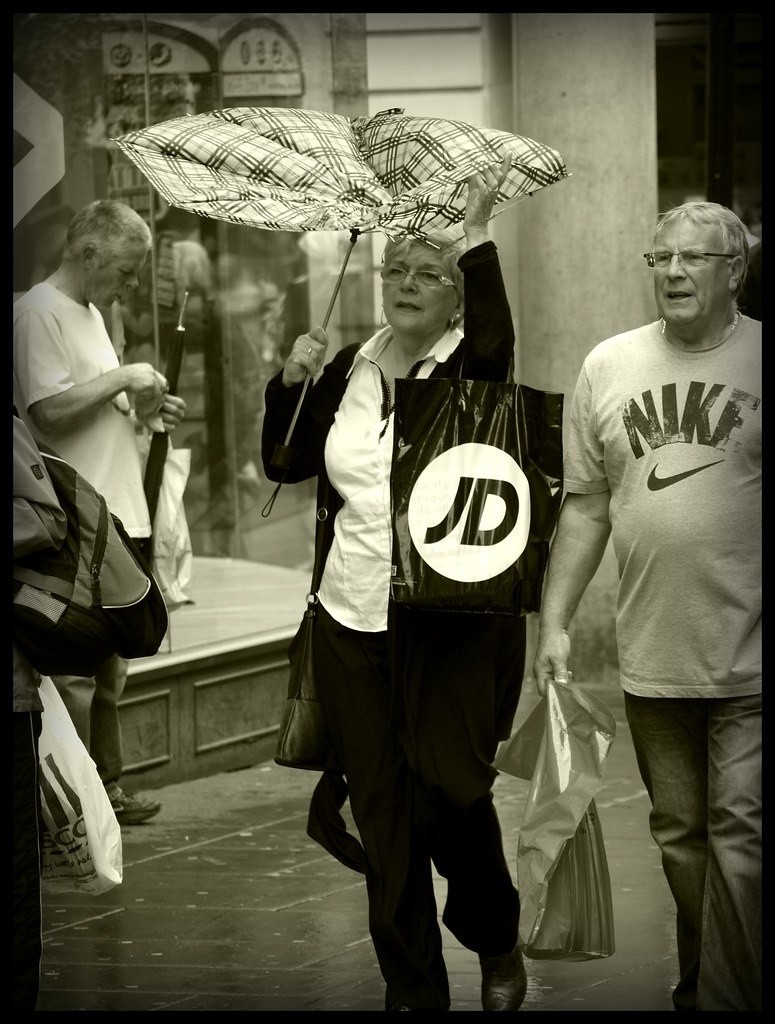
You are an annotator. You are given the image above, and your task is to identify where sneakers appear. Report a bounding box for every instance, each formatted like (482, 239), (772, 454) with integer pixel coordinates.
(109, 793), (162, 824)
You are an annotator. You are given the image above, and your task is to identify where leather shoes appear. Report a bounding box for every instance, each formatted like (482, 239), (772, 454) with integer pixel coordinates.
(479, 942), (527, 1010)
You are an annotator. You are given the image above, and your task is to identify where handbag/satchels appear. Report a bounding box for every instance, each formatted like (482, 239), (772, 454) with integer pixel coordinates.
(489, 678), (616, 962)
(14, 408), (168, 676)
(39, 676), (122, 895)
(135, 413), (192, 614)
(274, 605), (343, 771)
(391, 350), (564, 614)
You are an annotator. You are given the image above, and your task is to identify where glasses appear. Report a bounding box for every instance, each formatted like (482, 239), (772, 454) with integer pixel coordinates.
(644, 251), (735, 269)
(382, 266), (457, 289)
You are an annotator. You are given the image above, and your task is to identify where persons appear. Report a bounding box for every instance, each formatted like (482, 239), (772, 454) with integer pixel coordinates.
(262, 158), (527, 1011)
(132, 209), (371, 493)
(534, 202), (762, 1010)
(13, 199), (188, 1010)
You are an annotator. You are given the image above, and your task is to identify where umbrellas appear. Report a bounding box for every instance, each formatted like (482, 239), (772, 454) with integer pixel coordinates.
(144, 325), (186, 527)
(110, 107), (573, 466)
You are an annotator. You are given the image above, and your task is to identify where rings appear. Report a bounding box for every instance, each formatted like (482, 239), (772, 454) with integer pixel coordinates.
(488, 186), (498, 195)
(308, 348), (312, 355)
